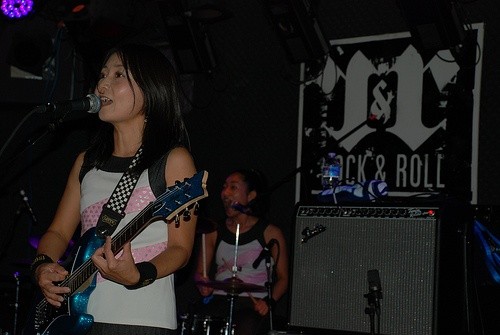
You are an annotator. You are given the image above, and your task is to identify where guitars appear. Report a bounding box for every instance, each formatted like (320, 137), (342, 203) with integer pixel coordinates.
(27, 169), (210, 335)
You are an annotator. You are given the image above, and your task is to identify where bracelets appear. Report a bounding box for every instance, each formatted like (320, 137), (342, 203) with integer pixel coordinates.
(125, 261), (157, 290)
(263, 295), (276, 308)
(30, 254), (54, 272)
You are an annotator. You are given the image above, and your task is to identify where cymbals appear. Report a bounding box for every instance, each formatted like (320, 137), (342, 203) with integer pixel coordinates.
(194, 278), (267, 294)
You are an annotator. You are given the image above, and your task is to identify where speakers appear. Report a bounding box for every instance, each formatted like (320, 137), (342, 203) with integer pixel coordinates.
(289, 204), (441, 335)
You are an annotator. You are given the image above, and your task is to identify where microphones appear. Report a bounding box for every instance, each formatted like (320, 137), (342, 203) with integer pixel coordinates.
(44, 94), (101, 113)
(231, 199), (260, 217)
(366, 268), (383, 307)
(252, 239), (275, 269)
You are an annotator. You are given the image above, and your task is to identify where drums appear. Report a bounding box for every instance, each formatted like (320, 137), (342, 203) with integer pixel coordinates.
(169, 311), (235, 335)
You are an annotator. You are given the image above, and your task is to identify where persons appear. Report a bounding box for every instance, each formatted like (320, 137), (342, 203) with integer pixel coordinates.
(175, 167), (288, 335)
(32, 42), (198, 335)
